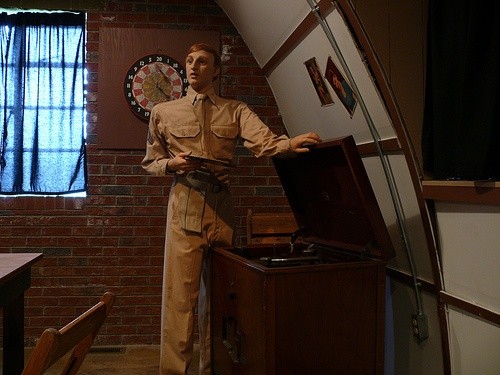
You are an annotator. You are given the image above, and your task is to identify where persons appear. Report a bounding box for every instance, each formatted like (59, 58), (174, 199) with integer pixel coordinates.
(307, 64), (358, 115)
(141, 42), (320, 375)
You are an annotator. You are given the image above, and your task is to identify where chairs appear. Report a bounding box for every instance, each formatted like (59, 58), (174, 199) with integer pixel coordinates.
(20, 292), (115, 375)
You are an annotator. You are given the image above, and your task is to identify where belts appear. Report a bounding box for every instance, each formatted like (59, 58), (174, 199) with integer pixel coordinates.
(175, 176), (230, 193)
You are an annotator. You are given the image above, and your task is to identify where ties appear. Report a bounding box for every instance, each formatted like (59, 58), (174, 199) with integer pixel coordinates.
(197, 94), (210, 160)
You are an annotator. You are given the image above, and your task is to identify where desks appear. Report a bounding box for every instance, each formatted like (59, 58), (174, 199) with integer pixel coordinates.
(0, 252), (44, 375)
